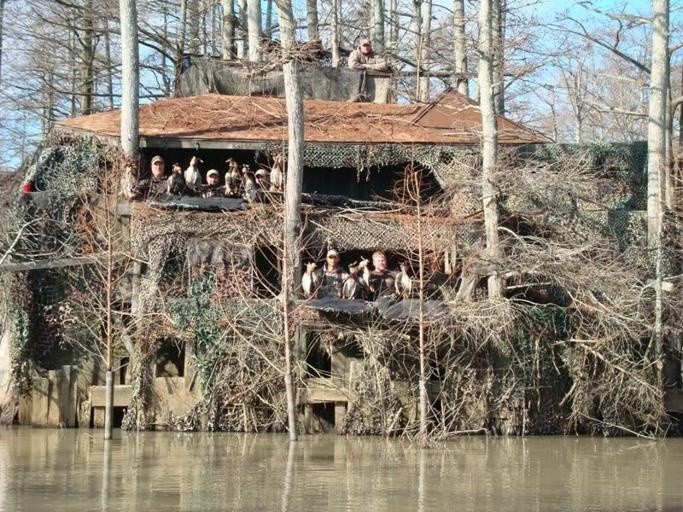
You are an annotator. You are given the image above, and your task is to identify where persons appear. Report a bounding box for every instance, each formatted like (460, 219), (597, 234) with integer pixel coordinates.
(348, 38), (389, 72)
(359, 249), (410, 301)
(187, 155), (238, 195)
(241, 155), (283, 196)
(306, 248), (356, 300)
(137, 152), (181, 194)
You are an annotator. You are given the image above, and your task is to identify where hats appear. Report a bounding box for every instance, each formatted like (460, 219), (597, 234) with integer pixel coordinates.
(254, 168), (269, 177)
(361, 39), (370, 46)
(206, 169), (219, 177)
(327, 249), (338, 258)
(151, 155), (165, 164)
